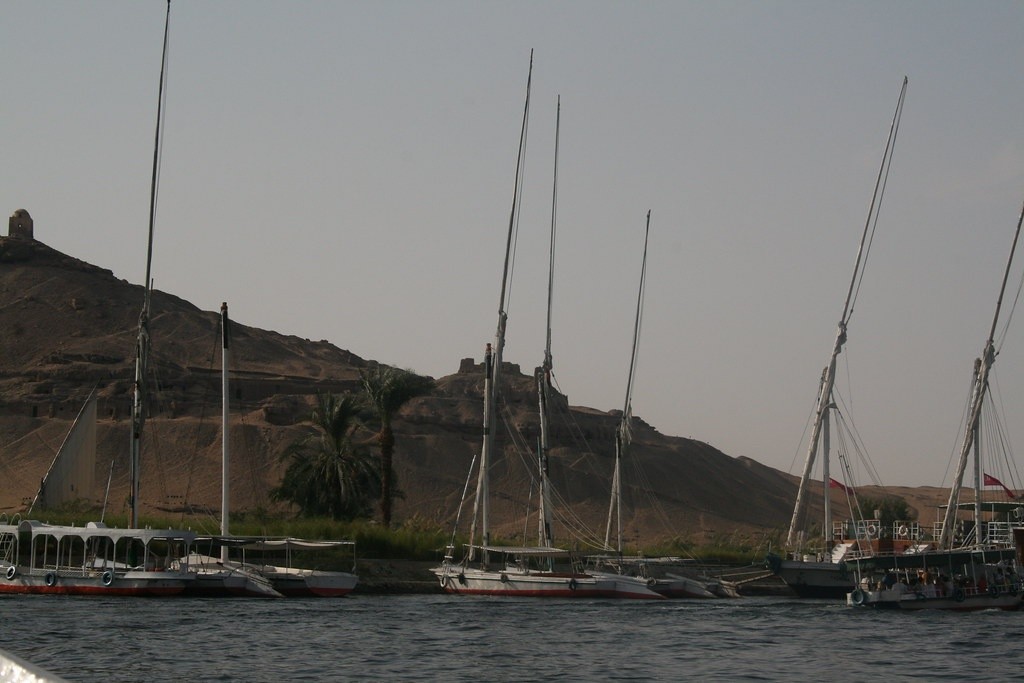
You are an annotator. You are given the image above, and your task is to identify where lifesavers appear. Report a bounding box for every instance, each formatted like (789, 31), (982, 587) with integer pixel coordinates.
(989, 585), (997, 597)
(501, 574), (507, 583)
(851, 589), (865, 604)
(1009, 585), (1017, 596)
(569, 578), (576, 591)
(102, 571), (113, 586)
(867, 524), (876, 535)
(459, 573), (464, 583)
(647, 577), (656, 586)
(45, 572), (57, 586)
(6, 566), (16, 579)
(954, 589), (964, 601)
(898, 525), (908, 535)
(440, 574), (448, 587)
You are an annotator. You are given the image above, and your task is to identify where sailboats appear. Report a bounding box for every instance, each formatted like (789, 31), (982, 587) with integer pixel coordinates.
(428, 49), (718, 601)
(842, 211), (1024, 614)
(722, 75), (925, 599)
(0, 1), (365, 600)
